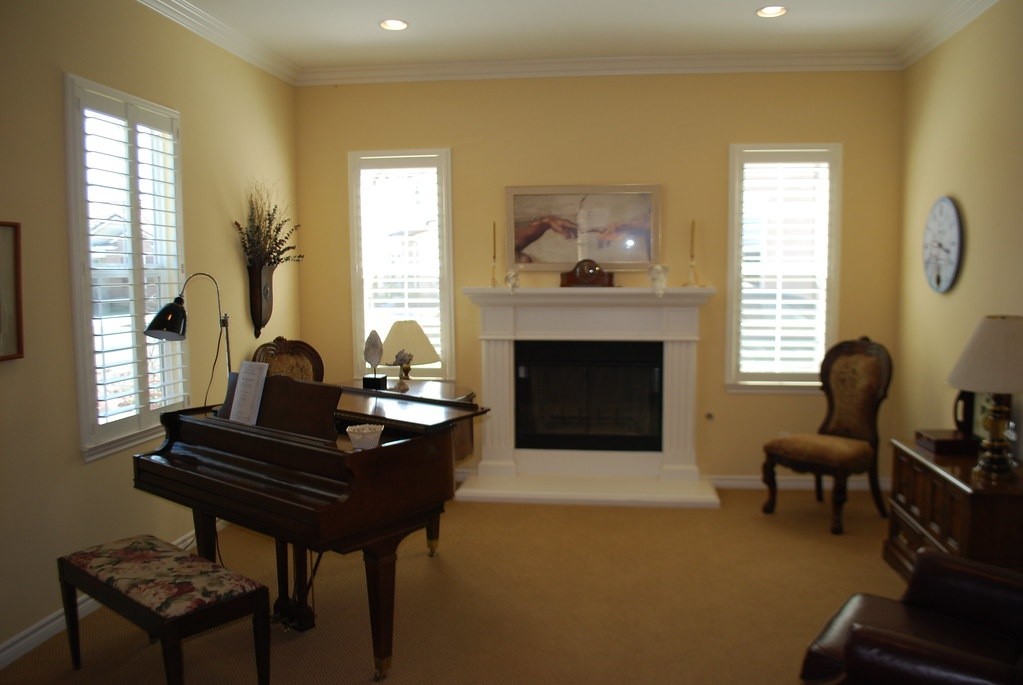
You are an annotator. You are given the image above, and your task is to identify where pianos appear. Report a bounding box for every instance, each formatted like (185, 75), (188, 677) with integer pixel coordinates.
(129, 370), (493, 680)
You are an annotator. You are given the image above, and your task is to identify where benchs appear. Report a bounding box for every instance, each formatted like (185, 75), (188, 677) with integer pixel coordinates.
(57, 533), (272, 685)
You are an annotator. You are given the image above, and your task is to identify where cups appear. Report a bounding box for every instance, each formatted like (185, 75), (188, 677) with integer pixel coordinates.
(504, 267), (519, 287)
(647, 263), (668, 290)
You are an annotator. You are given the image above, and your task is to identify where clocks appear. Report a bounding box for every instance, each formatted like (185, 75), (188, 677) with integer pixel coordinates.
(922, 196), (964, 293)
(946, 314), (1023, 482)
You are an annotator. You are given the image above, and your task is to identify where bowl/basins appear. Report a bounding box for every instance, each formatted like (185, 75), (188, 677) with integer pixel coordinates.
(346, 424), (384, 450)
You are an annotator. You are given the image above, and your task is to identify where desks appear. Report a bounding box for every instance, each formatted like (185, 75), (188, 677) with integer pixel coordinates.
(341, 378), (476, 464)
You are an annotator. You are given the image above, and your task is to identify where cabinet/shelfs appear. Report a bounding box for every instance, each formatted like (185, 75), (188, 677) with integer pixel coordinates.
(881, 437), (1023, 582)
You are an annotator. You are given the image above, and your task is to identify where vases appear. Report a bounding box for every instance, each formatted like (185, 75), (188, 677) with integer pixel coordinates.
(246, 264), (278, 337)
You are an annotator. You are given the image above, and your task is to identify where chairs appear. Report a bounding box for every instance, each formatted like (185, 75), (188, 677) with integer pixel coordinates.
(801, 547), (1023, 685)
(762, 335), (893, 535)
(253, 336), (324, 382)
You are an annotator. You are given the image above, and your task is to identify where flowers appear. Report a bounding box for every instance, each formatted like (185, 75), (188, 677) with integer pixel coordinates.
(235, 185), (305, 265)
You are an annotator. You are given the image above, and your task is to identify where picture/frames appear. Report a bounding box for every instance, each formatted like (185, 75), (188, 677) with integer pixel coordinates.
(0, 220), (25, 361)
(505, 184), (663, 272)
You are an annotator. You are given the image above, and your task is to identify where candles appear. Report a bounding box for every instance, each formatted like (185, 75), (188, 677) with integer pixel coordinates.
(690, 218), (695, 260)
(492, 220), (497, 260)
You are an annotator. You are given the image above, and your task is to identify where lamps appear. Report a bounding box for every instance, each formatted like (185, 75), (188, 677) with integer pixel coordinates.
(142, 273), (231, 403)
(380, 320), (441, 380)
